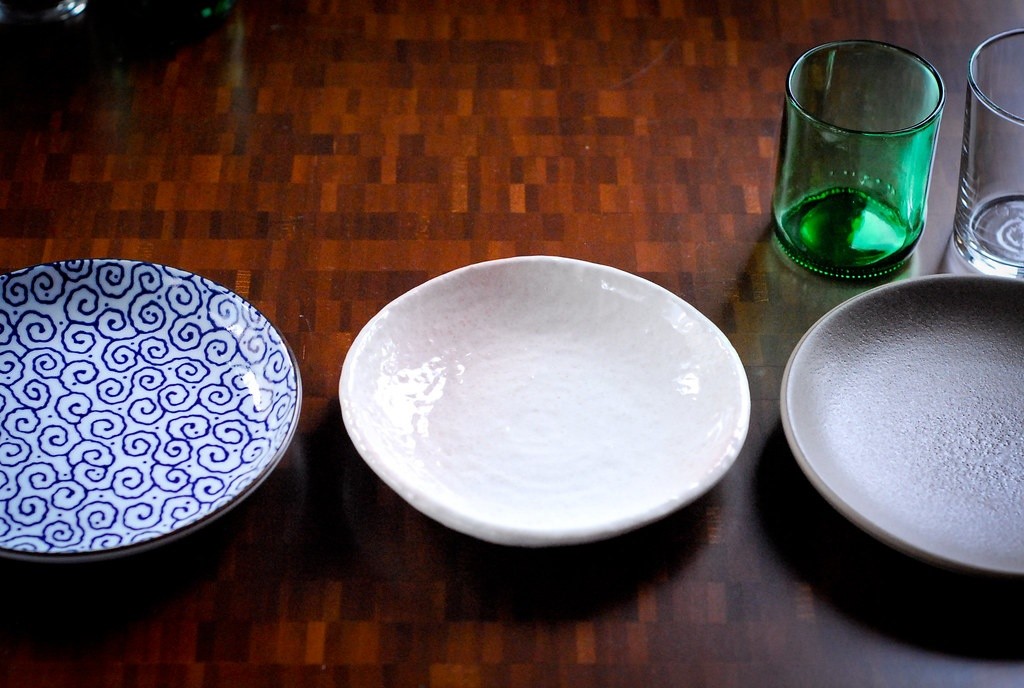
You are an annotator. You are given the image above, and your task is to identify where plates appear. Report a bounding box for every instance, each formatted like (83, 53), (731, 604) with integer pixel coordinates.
(0, 259), (303, 565)
(778, 271), (1024, 576)
(337, 253), (752, 548)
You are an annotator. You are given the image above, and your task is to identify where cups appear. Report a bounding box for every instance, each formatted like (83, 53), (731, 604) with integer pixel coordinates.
(769, 39), (946, 278)
(952, 28), (1024, 279)
